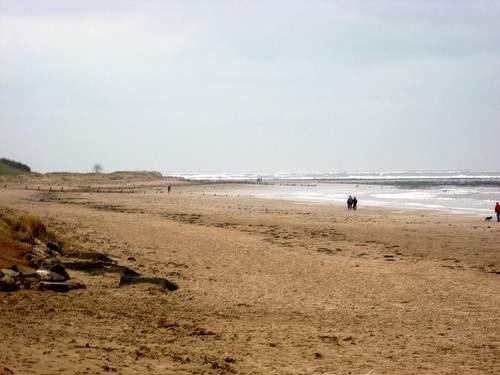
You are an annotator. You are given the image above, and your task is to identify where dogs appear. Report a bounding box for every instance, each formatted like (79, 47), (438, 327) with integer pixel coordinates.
(485, 217), (493, 222)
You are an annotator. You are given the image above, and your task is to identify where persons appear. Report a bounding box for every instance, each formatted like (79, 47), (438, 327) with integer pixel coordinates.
(495, 202), (500, 222)
(352, 197), (357, 210)
(168, 185), (170, 193)
(347, 196), (354, 210)
